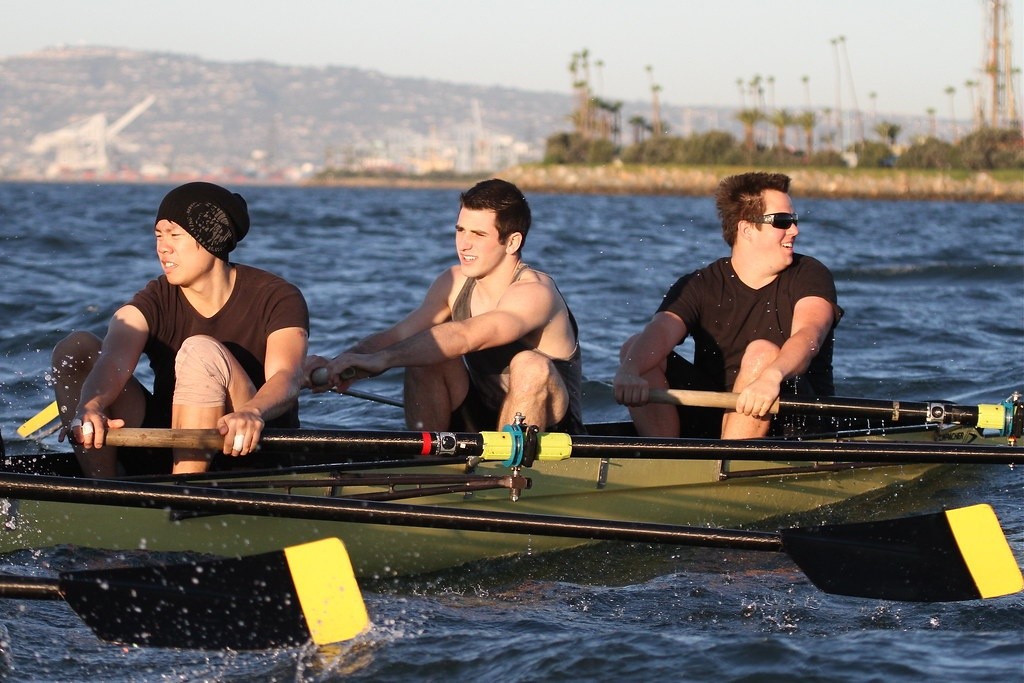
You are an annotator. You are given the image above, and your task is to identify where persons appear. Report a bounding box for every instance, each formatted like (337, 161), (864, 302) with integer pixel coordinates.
(50, 181), (310, 479)
(611, 173), (845, 439)
(302, 179), (586, 437)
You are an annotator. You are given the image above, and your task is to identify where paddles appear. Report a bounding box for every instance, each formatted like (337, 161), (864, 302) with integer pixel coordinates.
(0, 536), (377, 651)
(16, 361), (359, 441)
(647, 385), (1024, 439)
(103, 426), (1024, 465)
(0, 471), (1024, 606)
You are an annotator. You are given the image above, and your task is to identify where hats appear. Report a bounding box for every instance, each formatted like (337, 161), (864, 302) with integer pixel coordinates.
(156, 182), (249, 261)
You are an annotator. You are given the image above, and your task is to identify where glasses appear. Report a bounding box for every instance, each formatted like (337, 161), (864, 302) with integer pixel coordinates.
(747, 209), (799, 230)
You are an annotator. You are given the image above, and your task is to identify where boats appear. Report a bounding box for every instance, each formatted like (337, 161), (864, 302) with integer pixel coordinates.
(0, 416), (1024, 573)
